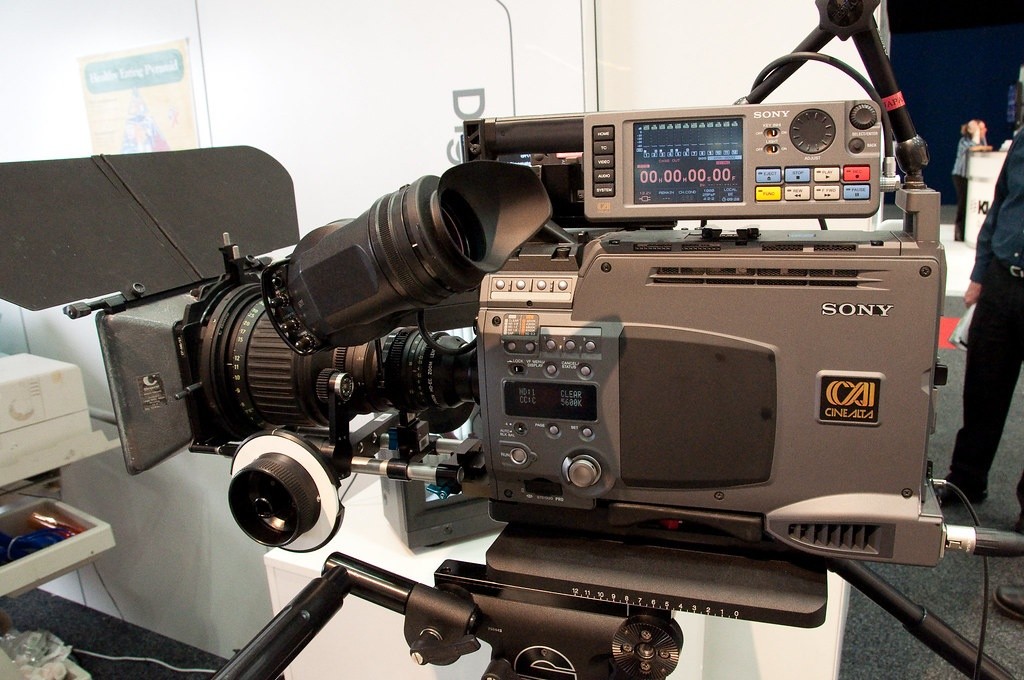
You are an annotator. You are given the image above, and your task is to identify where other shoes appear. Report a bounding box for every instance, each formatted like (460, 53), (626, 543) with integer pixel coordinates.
(1015, 510), (1024, 532)
(955, 224), (964, 240)
(935, 474), (986, 503)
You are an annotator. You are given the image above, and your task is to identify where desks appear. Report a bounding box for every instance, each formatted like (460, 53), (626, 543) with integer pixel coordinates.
(0, 415), (850, 680)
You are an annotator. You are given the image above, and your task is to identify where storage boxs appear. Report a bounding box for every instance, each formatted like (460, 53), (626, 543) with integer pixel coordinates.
(0, 498), (116, 598)
(0, 352), (92, 468)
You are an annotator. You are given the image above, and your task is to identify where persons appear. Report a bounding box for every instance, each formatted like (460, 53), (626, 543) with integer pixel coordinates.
(951, 120), (990, 242)
(932, 130), (1024, 537)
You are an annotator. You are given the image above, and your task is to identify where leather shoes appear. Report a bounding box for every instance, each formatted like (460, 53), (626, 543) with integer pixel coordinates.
(993, 584), (1024, 622)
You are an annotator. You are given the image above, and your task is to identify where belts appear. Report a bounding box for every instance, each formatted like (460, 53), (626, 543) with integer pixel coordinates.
(991, 257), (1024, 282)
